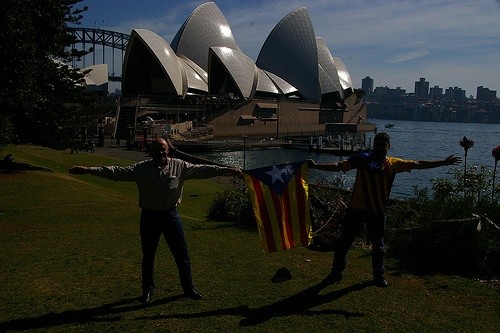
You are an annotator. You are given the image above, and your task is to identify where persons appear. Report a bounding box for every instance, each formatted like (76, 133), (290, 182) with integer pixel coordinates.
(70, 141), (96, 154)
(69, 138), (244, 303)
(307, 132), (463, 287)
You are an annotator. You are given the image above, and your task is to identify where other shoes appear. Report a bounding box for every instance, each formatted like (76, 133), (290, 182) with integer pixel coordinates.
(374, 278), (388, 287)
(326, 273), (343, 282)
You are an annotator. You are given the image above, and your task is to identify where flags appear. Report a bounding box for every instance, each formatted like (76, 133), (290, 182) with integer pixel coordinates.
(242, 161), (314, 256)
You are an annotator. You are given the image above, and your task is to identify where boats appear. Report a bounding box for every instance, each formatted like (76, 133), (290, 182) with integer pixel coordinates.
(384, 121), (397, 129)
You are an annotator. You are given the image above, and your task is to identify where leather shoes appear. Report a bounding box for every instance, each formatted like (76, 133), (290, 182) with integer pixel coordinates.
(184, 290), (202, 299)
(142, 290), (151, 303)
